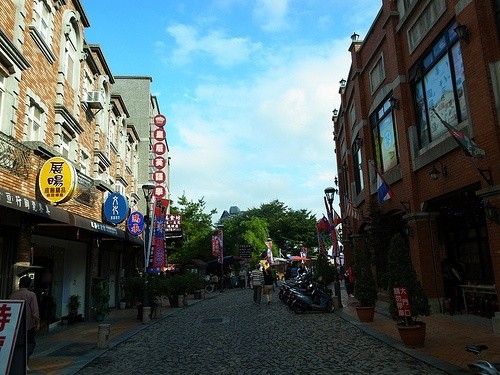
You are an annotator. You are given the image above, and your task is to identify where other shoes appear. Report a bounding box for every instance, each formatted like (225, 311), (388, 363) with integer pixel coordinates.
(266, 300), (270, 305)
(349, 294), (354, 298)
(252, 299), (260, 305)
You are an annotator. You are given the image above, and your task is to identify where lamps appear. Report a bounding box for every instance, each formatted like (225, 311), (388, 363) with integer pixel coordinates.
(453, 22), (471, 44)
(339, 78), (346, 86)
(350, 32), (359, 40)
(388, 95), (401, 111)
(428, 159), (447, 179)
(335, 177), (339, 195)
(333, 109), (338, 115)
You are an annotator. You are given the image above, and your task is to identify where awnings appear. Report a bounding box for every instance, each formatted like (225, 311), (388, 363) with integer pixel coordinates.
(0, 188), (145, 250)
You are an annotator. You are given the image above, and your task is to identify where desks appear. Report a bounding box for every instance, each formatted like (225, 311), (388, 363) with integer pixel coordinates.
(459, 284), (496, 314)
(61, 314), (82, 328)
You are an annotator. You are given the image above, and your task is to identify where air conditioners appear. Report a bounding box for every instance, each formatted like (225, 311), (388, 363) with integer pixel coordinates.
(87, 90), (105, 109)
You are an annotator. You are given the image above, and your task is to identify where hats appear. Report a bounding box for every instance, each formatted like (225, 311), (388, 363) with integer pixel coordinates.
(255, 264), (260, 267)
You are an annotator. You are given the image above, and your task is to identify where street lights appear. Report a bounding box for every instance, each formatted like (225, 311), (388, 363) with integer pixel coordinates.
(323, 187), (344, 308)
(139, 184), (157, 320)
(217, 224), (224, 293)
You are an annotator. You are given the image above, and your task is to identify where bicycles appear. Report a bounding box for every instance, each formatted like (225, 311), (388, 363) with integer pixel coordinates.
(205, 274), (217, 293)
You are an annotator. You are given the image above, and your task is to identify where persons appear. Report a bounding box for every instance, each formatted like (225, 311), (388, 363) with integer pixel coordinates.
(10, 276), (40, 371)
(239, 264), (279, 306)
(284, 264), (312, 282)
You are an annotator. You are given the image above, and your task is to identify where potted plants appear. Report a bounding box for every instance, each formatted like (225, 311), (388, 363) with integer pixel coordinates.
(92, 287), (113, 322)
(347, 236), (377, 323)
(120, 270), (207, 320)
(68, 295), (80, 315)
(386, 232), (431, 349)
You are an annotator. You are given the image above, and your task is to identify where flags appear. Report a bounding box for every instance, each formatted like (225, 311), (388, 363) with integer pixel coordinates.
(318, 217), (330, 233)
(377, 173), (395, 206)
(334, 212), (341, 228)
(347, 198), (360, 220)
(441, 120), (486, 158)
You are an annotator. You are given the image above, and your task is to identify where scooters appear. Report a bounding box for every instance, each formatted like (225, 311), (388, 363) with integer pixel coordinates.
(279, 274), (333, 315)
